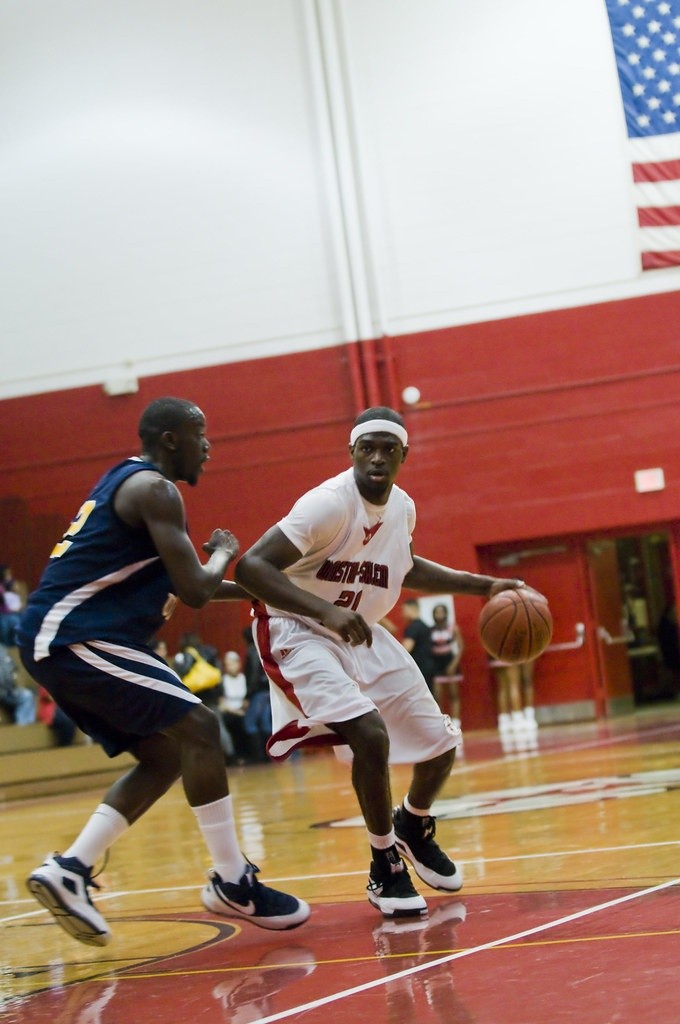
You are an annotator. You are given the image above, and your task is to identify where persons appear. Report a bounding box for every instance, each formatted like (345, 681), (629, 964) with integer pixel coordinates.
(234, 405), (547, 918)
(149, 637), (167, 659)
(429, 604), (463, 675)
(0, 564), (27, 647)
(0, 643), (36, 725)
(217, 649), (249, 764)
(184, 629), (235, 766)
(238, 623), (273, 766)
(401, 598), (434, 697)
(15, 398), (311, 946)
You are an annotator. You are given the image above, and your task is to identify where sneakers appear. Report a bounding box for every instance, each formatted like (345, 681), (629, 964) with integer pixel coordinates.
(391, 804), (463, 893)
(417, 902), (467, 971)
(201, 851), (310, 931)
(213, 947), (315, 1018)
(371, 916), (429, 966)
(366, 858), (429, 917)
(27, 850), (112, 947)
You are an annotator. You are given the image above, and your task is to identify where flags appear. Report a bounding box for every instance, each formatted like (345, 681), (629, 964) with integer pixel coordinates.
(605, 1), (679, 270)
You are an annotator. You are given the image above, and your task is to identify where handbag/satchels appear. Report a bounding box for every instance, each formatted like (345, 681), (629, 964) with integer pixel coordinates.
(182, 646), (221, 692)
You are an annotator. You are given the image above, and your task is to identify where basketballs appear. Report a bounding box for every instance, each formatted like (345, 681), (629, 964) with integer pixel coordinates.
(481, 586), (557, 668)
